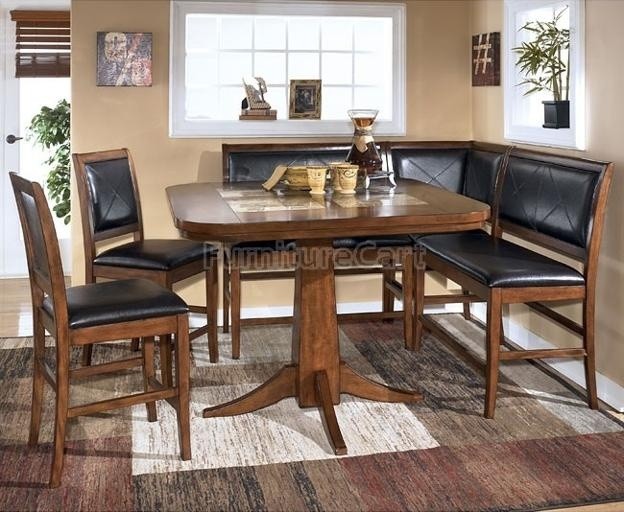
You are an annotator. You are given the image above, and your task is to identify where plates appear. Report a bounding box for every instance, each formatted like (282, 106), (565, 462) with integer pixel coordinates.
(280, 179), (331, 191)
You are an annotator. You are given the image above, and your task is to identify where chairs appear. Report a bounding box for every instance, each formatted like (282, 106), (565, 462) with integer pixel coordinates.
(6, 172), (203, 489)
(71, 147), (222, 388)
(383, 138), (511, 350)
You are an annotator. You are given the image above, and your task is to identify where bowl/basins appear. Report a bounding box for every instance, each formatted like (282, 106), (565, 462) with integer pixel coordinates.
(285, 165), (328, 186)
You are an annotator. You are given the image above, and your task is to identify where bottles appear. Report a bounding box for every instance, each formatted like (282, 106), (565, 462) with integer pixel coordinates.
(344, 109), (383, 174)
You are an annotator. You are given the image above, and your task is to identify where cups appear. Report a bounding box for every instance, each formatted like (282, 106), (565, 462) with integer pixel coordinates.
(337, 164), (359, 192)
(306, 166), (328, 193)
(329, 162), (351, 190)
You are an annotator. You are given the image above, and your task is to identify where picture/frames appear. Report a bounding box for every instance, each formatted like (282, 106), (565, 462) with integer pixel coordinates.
(288, 79), (322, 120)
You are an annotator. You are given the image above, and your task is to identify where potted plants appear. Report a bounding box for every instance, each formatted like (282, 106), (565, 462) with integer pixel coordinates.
(511, 8), (574, 128)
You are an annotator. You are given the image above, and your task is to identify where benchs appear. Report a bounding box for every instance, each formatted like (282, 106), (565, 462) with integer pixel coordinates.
(221, 139), (415, 359)
(413, 146), (615, 418)
